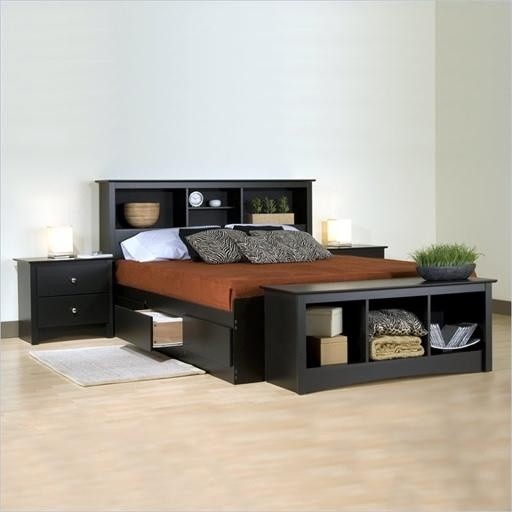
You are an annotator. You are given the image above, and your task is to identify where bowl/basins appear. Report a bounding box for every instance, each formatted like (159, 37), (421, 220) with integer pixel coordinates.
(208, 200), (222, 207)
(123, 202), (161, 228)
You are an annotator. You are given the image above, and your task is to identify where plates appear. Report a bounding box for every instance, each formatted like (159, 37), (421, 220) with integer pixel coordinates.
(189, 191), (203, 207)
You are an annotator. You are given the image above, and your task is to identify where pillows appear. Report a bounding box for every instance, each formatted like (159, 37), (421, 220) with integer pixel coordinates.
(364, 305), (432, 339)
(121, 224), (331, 264)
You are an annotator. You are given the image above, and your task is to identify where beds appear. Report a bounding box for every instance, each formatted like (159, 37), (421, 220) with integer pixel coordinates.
(93, 176), (477, 384)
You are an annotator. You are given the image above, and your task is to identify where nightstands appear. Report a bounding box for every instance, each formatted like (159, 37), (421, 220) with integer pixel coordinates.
(324, 240), (388, 259)
(12, 253), (117, 346)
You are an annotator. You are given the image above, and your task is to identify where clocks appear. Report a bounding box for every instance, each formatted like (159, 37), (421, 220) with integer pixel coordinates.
(189, 191), (205, 209)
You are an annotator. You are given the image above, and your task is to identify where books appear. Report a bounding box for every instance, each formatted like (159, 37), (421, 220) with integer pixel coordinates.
(429, 321), (477, 348)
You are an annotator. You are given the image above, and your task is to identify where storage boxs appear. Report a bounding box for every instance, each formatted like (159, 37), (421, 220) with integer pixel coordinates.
(304, 304), (347, 337)
(306, 334), (349, 367)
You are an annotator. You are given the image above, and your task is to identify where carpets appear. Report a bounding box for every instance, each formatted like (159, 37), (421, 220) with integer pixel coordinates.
(27, 339), (208, 386)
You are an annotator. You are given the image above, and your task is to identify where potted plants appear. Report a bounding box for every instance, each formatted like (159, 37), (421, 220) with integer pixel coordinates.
(407, 242), (484, 282)
(246, 194), (297, 225)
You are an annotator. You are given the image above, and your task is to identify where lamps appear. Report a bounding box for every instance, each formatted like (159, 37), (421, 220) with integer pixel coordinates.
(326, 216), (353, 248)
(43, 225), (74, 259)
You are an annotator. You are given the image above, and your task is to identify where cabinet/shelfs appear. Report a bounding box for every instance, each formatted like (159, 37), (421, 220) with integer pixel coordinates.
(256, 274), (499, 397)
